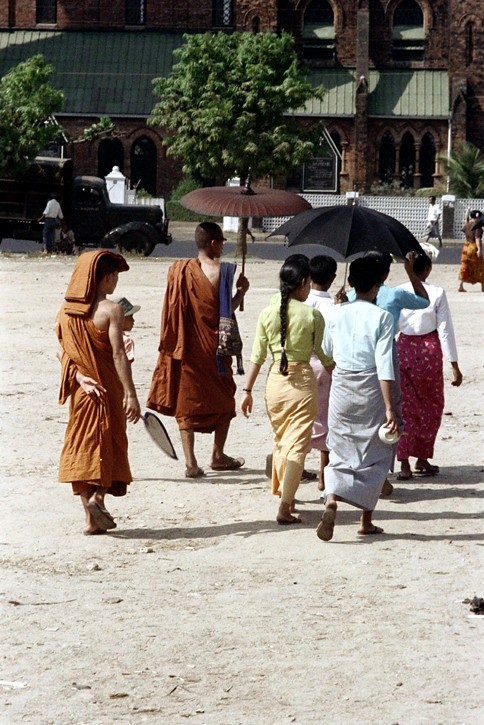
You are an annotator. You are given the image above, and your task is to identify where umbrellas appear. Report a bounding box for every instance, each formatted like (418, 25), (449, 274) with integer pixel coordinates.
(180, 171), (312, 313)
(261, 197), (428, 293)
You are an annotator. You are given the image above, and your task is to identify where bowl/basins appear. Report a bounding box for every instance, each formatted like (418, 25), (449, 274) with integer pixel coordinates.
(378, 423), (402, 444)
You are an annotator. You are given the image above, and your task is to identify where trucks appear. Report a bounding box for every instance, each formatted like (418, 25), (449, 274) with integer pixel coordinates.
(0, 156), (173, 256)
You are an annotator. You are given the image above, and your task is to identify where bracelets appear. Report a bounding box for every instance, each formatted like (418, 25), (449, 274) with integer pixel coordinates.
(243, 388), (253, 393)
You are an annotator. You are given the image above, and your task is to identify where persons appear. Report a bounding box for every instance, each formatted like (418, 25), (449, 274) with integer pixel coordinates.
(239, 258), (336, 525)
(303, 254), (349, 490)
(387, 243), (464, 481)
(49, 248), (139, 535)
(425, 194), (444, 251)
(36, 193), (76, 254)
(147, 223), (253, 480)
(458, 210), (484, 294)
(281, 251), (319, 483)
(109, 297), (141, 369)
(316, 256), (406, 543)
(344, 250), (434, 497)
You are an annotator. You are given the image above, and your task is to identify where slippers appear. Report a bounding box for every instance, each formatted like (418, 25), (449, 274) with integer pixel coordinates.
(84, 529), (106, 534)
(186, 468), (207, 477)
(277, 465), (439, 540)
(212, 457), (245, 470)
(87, 502), (117, 529)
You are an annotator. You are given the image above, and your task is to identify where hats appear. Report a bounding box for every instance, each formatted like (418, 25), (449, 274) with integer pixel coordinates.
(114, 297), (140, 318)
